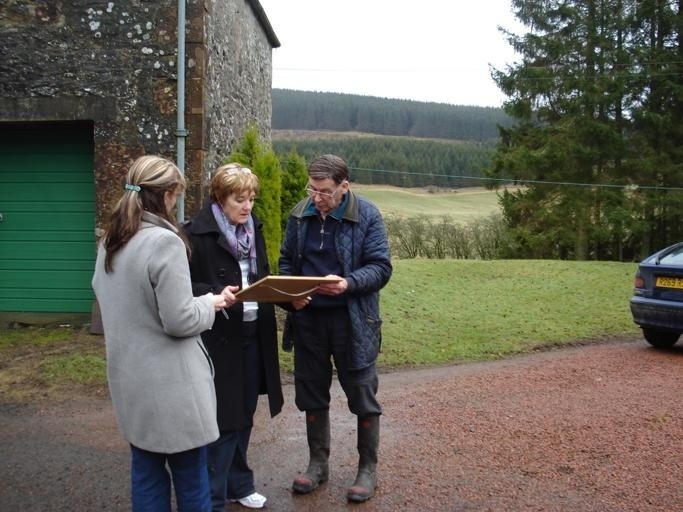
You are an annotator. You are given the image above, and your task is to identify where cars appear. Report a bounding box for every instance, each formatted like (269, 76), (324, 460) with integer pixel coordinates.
(629, 242), (683, 351)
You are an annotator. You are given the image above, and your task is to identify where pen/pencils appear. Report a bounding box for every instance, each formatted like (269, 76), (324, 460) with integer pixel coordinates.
(211, 287), (230, 320)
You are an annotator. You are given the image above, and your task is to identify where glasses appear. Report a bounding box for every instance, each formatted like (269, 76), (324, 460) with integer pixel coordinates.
(305, 178), (346, 200)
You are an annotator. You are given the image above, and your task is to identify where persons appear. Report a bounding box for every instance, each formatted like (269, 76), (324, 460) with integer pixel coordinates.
(176, 164), (312, 511)
(278, 155), (393, 503)
(90, 154), (226, 511)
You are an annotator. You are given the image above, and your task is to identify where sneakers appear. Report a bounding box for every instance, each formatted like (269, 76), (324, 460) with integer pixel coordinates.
(230, 491), (267, 508)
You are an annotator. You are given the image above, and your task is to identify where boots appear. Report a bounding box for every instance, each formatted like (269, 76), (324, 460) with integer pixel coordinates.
(292, 409), (330, 491)
(347, 416), (380, 501)
